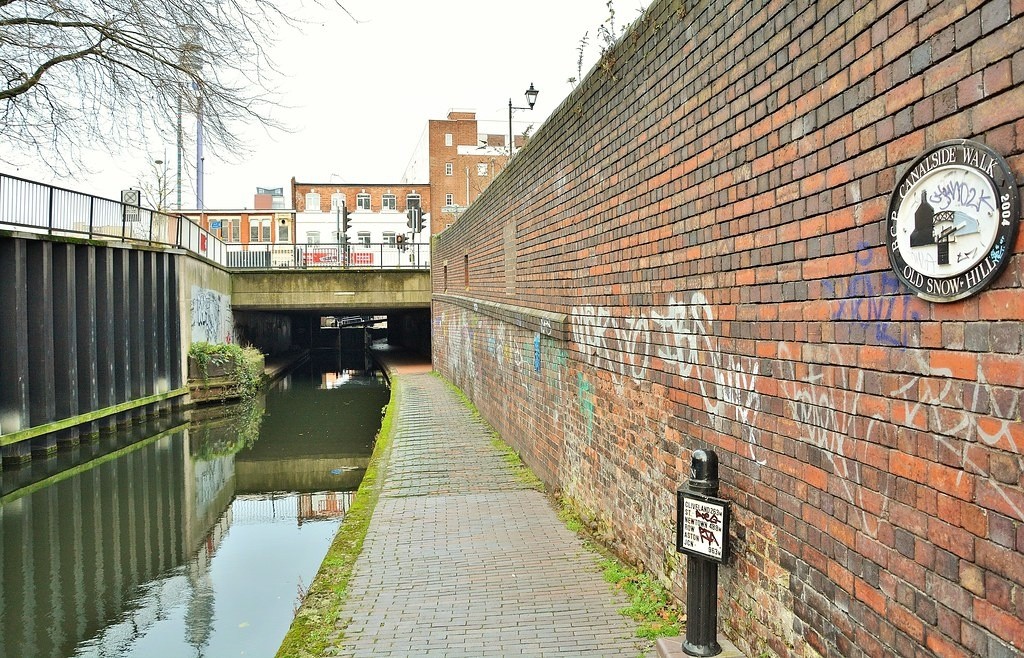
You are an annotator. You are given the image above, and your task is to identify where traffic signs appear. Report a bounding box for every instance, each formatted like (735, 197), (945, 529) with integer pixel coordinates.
(212, 222), (220, 229)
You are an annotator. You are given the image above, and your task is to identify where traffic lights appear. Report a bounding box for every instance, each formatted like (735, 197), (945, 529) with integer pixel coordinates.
(397, 235), (402, 249)
(343, 206), (352, 232)
(418, 208), (427, 234)
(345, 234), (351, 252)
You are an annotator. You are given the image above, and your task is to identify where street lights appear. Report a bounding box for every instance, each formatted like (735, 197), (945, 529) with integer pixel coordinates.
(200, 157), (205, 228)
(154, 148), (168, 243)
(508, 82), (539, 159)
(297, 495), (303, 530)
(271, 493), (278, 523)
